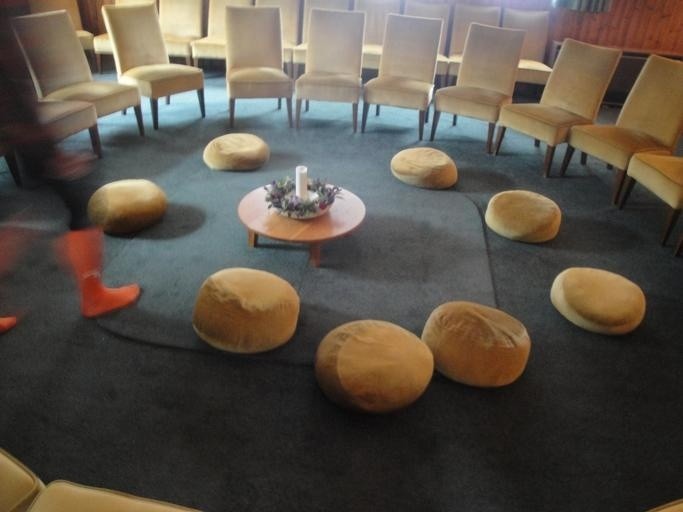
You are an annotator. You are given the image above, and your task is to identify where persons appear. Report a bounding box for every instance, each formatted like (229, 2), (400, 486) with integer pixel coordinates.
(0, 48), (142, 332)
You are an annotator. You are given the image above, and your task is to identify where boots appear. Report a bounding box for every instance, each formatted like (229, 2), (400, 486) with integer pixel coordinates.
(64, 225), (140, 318)
(0, 316), (17, 333)
